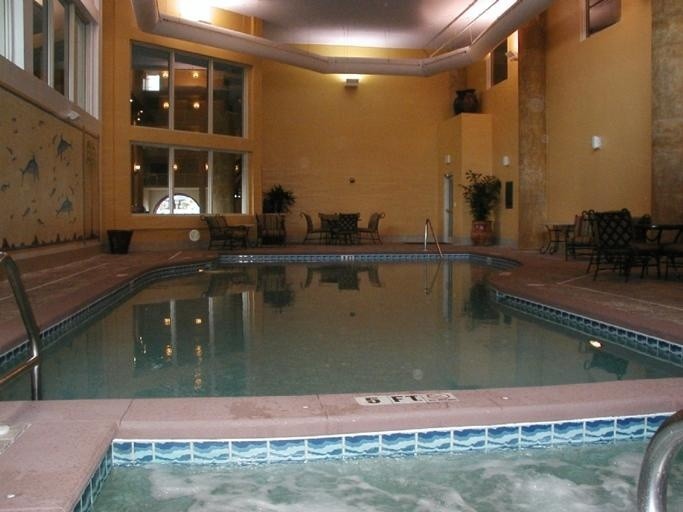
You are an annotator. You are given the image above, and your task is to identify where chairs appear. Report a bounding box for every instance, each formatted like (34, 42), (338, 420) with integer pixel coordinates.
(201, 212), (250, 250)
(302, 265), (380, 291)
(301, 209), (385, 246)
(541, 208), (682, 283)
(198, 271), (256, 300)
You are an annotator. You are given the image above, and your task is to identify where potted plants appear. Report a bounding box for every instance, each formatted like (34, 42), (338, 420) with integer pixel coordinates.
(258, 183), (297, 236)
(458, 169), (502, 246)
(262, 272), (294, 315)
